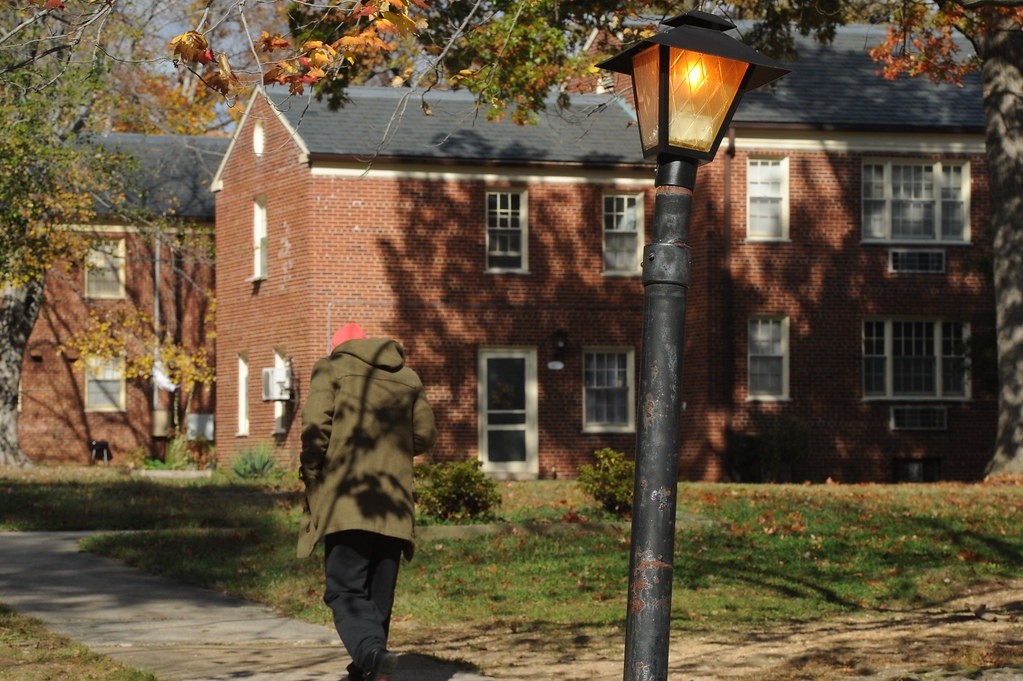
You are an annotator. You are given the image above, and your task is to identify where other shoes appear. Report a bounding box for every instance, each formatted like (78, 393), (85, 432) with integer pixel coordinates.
(347, 662), (364, 681)
(363, 648), (398, 681)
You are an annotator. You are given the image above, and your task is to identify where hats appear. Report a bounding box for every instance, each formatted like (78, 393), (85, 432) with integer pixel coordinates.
(330, 323), (368, 354)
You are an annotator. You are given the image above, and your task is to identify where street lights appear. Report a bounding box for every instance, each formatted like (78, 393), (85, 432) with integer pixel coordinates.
(593, 10), (788, 680)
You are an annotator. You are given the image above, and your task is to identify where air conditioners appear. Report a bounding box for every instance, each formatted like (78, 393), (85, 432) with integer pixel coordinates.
(261, 367), (293, 402)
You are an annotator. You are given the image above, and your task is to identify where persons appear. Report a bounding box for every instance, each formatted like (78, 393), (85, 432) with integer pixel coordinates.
(295, 323), (438, 681)
